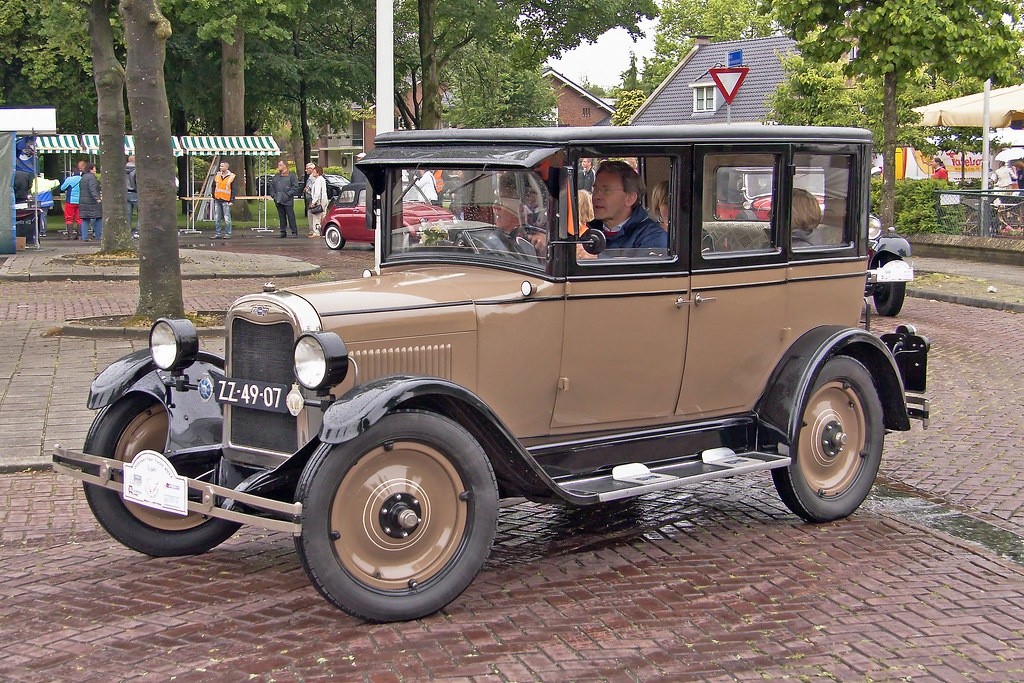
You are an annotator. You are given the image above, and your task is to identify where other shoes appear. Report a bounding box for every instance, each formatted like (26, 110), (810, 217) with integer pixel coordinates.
(308, 234), (320, 238)
(223, 234), (232, 239)
(209, 234), (222, 239)
(39, 233), (46, 236)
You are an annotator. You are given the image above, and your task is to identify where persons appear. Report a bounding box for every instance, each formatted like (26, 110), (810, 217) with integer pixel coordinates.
(1015, 158), (1024, 229)
(58, 160), (103, 243)
(929, 157), (948, 235)
(13, 170), (41, 245)
(209, 160), (237, 239)
(268, 158), (299, 239)
(758, 187), (824, 252)
(413, 169), (465, 221)
(527, 157), (670, 260)
(302, 162), (329, 238)
(991, 160), (1017, 236)
(30, 171), (61, 237)
(350, 152), (367, 184)
(125, 155), (140, 239)
(174, 165), (182, 237)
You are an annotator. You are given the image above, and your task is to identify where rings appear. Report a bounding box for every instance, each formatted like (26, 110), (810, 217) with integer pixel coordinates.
(578, 253), (582, 258)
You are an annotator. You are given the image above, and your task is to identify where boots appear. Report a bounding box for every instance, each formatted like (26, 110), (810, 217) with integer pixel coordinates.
(74, 224), (82, 240)
(65, 224), (73, 240)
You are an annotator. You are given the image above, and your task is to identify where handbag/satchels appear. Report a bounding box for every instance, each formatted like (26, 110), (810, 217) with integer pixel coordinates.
(311, 204), (324, 214)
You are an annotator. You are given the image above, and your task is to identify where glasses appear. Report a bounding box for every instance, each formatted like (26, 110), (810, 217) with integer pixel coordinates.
(589, 185), (625, 195)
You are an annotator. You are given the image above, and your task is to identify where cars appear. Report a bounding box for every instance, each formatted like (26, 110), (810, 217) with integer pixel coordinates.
(715, 167), (914, 318)
(296, 173), (352, 199)
(318, 182), (456, 250)
(49, 122), (930, 624)
(254, 174), (275, 195)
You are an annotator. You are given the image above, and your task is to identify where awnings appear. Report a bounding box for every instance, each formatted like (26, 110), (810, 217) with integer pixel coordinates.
(29, 133), (102, 157)
(123, 134), (281, 156)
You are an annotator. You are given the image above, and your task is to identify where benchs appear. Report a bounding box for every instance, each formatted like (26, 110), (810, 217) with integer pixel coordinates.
(704, 219), (842, 250)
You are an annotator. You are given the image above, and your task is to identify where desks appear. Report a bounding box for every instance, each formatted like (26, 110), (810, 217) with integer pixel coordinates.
(176, 194), (273, 234)
(17, 203), (41, 249)
(28, 194), (94, 235)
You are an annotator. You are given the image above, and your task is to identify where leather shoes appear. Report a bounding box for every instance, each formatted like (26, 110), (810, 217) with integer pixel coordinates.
(292, 233), (299, 238)
(276, 233), (287, 237)
